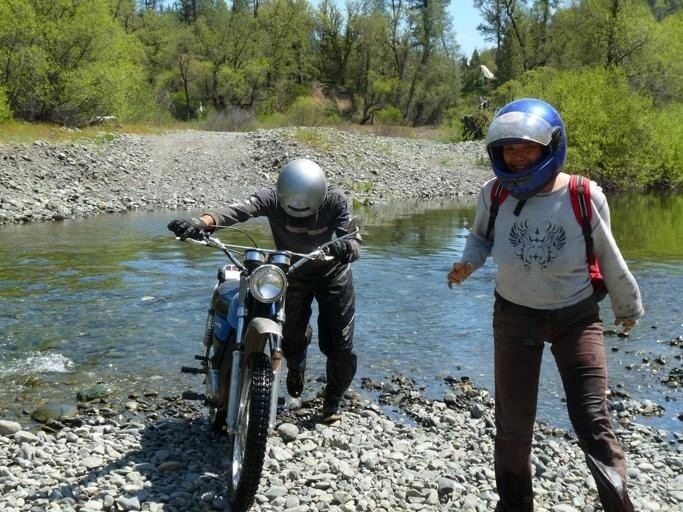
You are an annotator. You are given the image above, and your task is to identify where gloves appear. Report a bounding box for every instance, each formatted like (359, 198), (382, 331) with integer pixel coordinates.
(327, 241), (353, 263)
(168, 216), (209, 242)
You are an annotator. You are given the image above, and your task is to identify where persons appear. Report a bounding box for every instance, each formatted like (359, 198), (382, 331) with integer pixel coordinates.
(167, 158), (356, 415)
(447, 99), (645, 512)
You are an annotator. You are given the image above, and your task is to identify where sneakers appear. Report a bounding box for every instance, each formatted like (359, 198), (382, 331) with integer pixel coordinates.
(287, 370), (305, 398)
(320, 398), (344, 422)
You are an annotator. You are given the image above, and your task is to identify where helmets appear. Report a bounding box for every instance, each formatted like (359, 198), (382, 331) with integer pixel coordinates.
(276, 157), (327, 217)
(485, 99), (566, 200)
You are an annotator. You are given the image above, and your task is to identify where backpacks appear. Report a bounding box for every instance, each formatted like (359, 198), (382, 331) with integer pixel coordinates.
(487, 175), (608, 302)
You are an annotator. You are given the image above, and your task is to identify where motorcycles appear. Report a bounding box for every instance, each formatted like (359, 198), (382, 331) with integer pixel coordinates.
(175, 215), (360, 511)
